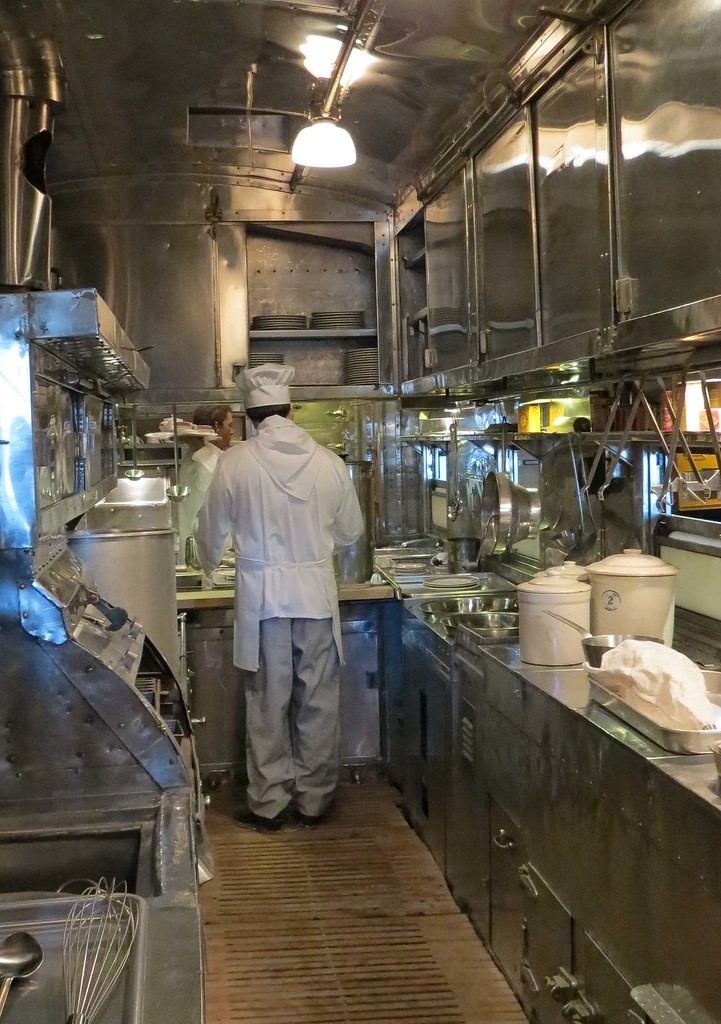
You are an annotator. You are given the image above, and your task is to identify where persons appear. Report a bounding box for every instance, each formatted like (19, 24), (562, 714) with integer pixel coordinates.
(194, 363), (365, 835)
(179, 405), (233, 565)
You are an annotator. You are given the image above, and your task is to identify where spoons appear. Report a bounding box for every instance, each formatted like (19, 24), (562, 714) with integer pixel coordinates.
(0, 931), (45, 1017)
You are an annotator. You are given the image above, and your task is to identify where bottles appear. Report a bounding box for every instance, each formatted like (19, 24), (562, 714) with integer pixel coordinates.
(662, 385), (673, 430)
(185, 533), (198, 569)
(158, 414), (184, 432)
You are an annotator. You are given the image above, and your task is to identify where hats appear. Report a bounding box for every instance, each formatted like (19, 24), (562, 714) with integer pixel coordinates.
(235, 361), (295, 409)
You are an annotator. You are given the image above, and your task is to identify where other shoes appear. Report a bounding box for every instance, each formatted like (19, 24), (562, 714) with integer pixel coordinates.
(297, 811), (317, 828)
(232, 810), (282, 833)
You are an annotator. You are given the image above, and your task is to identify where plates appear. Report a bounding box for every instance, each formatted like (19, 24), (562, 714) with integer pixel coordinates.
(422, 574), (480, 592)
(310, 309), (367, 330)
(390, 562), (430, 576)
(249, 352), (286, 369)
(365, 580), (389, 585)
(344, 348), (379, 385)
(252, 314), (308, 330)
(181, 432), (219, 437)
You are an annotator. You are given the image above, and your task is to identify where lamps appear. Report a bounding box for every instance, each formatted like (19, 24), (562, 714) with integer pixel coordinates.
(292, 105), (356, 168)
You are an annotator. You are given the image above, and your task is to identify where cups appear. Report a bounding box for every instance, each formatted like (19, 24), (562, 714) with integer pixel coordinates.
(173, 422), (215, 433)
(370, 571), (382, 583)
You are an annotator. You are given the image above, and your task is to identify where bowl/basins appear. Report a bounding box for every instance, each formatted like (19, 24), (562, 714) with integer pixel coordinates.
(212, 567), (236, 587)
(144, 432), (175, 444)
(420, 596), (519, 621)
(439, 611), (519, 639)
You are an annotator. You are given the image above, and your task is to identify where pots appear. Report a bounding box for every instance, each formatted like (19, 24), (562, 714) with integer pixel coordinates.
(516, 547), (682, 667)
(543, 609), (665, 669)
